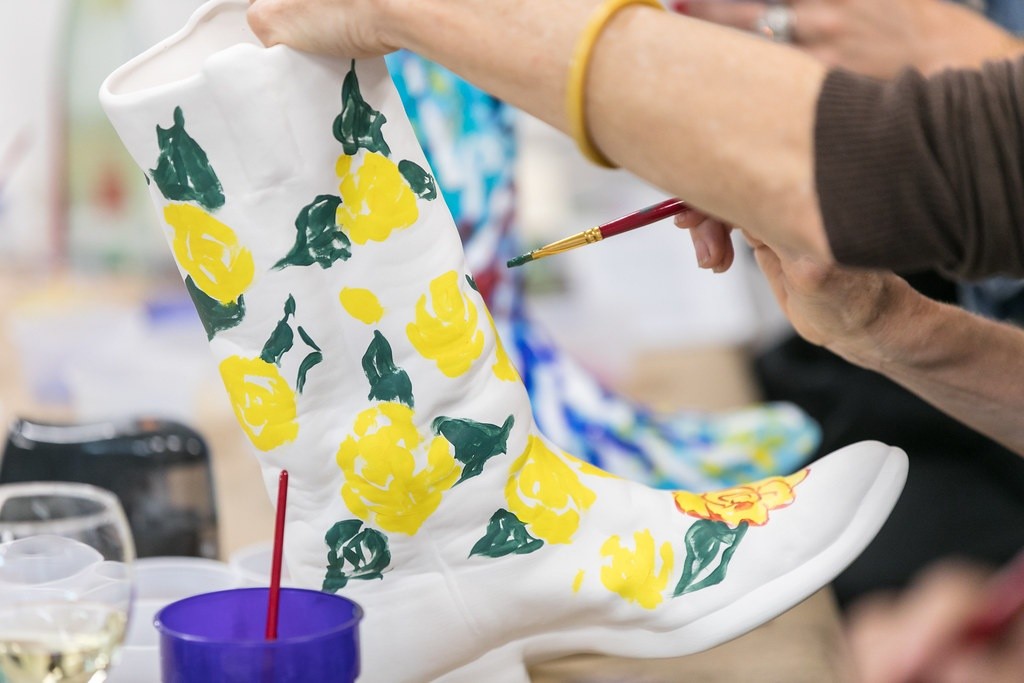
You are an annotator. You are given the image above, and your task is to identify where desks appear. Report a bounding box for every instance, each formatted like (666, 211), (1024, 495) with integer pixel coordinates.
(0, 272), (861, 683)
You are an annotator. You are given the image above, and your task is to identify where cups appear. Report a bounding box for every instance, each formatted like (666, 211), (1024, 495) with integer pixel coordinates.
(0, 482), (364, 683)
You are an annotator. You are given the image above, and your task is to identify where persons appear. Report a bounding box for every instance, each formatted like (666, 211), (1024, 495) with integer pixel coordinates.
(846, 555), (1024, 683)
(245, 0), (1024, 460)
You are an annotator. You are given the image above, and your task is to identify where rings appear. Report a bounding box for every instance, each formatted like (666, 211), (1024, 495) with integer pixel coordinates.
(756, 5), (795, 43)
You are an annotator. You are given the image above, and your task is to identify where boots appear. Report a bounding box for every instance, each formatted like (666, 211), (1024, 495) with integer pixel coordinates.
(383, 45), (822, 490)
(97, 0), (910, 683)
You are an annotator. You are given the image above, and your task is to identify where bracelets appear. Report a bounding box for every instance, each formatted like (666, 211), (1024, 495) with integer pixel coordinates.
(566, 0), (667, 170)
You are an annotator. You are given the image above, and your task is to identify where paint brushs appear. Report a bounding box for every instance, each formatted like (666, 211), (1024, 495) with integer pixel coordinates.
(503, 197), (690, 269)
(265, 466), (290, 640)
